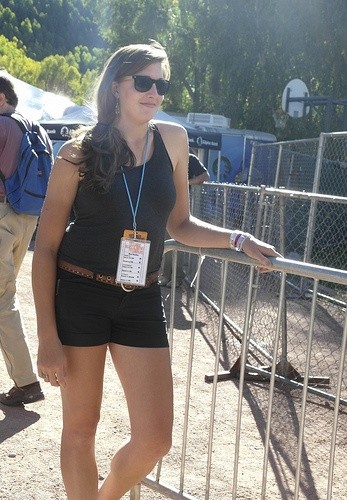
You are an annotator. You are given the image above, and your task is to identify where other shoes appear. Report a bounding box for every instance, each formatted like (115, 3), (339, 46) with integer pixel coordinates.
(158, 276), (182, 287)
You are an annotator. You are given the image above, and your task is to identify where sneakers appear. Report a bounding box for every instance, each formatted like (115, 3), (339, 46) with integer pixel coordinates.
(0, 382), (45, 405)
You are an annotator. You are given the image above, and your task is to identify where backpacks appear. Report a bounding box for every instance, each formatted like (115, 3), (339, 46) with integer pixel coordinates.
(0, 113), (53, 216)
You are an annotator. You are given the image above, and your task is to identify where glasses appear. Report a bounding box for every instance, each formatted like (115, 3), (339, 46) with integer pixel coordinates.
(120, 75), (171, 96)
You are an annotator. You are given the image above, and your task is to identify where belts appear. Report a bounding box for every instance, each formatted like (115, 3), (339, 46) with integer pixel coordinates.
(57, 260), (159, 292)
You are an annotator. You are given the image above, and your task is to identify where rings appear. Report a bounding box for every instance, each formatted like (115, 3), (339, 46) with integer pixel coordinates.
(42, 374), (49, 378)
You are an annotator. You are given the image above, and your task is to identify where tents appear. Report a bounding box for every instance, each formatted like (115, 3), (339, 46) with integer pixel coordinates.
(0, 67), (223, 185)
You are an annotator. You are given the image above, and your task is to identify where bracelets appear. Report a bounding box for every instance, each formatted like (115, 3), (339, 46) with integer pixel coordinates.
(229, 230), (252, 253)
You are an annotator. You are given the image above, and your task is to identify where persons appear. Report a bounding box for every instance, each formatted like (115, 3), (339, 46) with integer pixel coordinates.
(157, 153), (211, 288)
(31, 39), (285, 500)
(0, 76), (54, 406)
